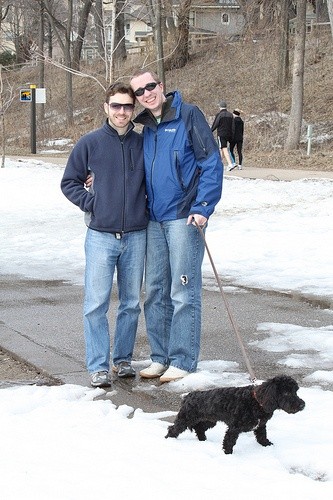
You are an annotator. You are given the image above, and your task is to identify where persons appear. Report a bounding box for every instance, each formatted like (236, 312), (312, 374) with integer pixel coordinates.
(60, 82), (150, 388)
(211, 101), (237, 172)
(85, 72), (223, 382)
(231, 108), (244, 170)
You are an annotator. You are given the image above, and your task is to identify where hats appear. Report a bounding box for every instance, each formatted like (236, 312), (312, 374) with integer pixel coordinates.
(219, 102), (227, 107)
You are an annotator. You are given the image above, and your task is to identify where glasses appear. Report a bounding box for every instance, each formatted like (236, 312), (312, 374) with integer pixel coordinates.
(109, 103), (134, 110)
(133, 81), (160, 96)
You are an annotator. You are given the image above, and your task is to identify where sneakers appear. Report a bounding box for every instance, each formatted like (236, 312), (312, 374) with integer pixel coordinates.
(112, 361), (136, 378)
(90, 371), (111, 387)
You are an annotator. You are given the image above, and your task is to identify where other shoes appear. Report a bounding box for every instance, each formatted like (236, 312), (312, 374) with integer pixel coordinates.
(234, 163), (237, 167)
(229, 168), (234, 171)
(238, 165), (242, 169)
(160, 366), (187, 381)
(140, 362), (167, 378)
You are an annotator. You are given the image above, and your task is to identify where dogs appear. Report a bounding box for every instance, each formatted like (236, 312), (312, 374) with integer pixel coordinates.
(165, 375), (305, 454)
